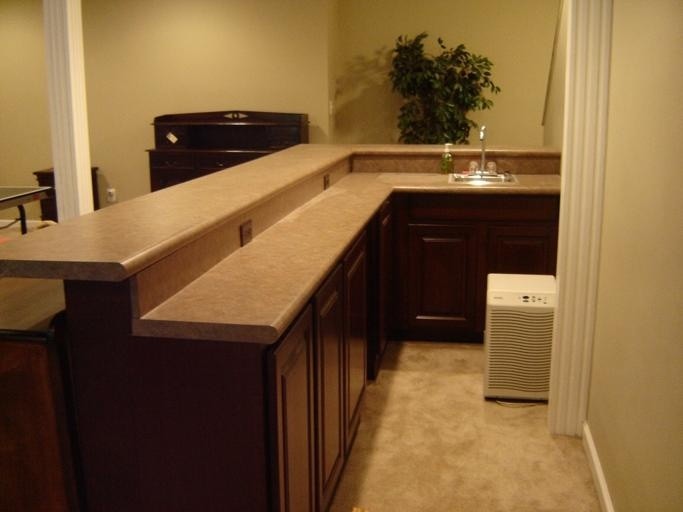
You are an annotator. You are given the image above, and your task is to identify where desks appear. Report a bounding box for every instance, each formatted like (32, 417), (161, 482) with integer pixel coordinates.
(0, 186), (52, 235)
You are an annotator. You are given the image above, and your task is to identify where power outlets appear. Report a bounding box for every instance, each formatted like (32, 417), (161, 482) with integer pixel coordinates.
(106, 188), (116, 202)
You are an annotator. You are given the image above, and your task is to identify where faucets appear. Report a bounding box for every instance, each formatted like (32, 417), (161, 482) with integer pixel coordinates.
(479, 126), (486, 172)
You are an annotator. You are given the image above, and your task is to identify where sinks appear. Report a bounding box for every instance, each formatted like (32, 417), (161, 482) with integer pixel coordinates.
(449, 173), (517, 185)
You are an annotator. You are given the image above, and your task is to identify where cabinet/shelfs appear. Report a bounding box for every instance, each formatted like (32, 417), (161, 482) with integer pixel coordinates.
(399, 194), (562, 342)
(0, 328), (88, 512)
(145, 110), (309, 193)
(63, 259), (349, 512)
(344, 187), (401, 452)
(32, 167), (100, 223)
